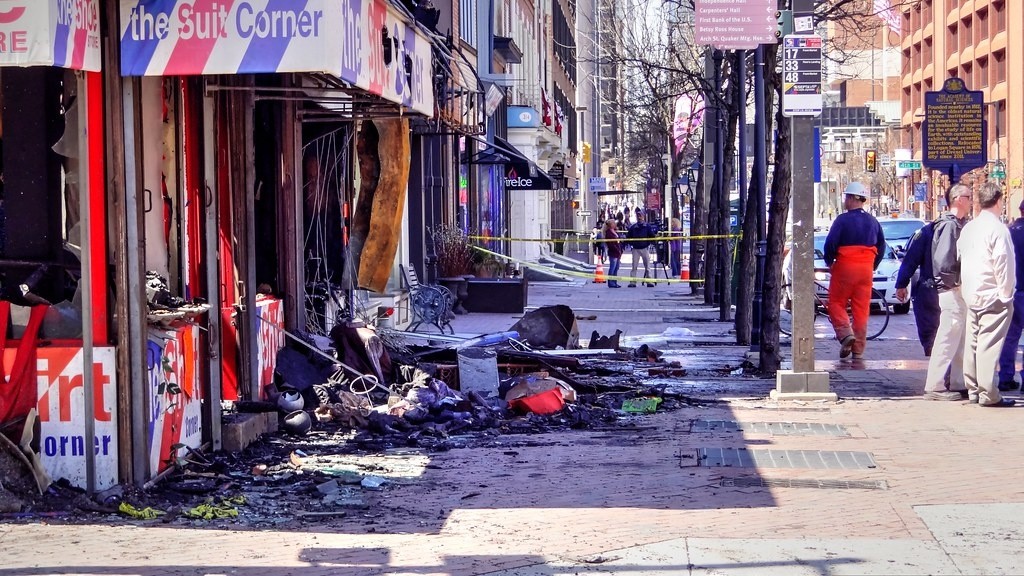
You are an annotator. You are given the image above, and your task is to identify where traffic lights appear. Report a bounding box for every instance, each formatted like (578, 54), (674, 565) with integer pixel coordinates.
(583, 142), (592, 163)
(864, 150), (877, 172)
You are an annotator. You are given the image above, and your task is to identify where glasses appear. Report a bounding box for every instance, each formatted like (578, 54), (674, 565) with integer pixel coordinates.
(952, 195), (972, 201)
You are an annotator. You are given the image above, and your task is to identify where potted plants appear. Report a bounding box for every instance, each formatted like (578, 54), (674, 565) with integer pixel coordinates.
(426, 222), (476, 320)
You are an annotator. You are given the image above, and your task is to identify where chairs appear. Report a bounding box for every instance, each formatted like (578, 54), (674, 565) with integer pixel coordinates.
(399, 263), (456, 335)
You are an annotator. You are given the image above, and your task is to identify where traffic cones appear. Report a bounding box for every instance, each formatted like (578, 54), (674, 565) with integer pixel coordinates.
(681, 253), (690, 280)
(593, 255), (608, 283)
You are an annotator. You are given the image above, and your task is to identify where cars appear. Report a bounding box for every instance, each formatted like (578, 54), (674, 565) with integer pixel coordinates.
(782, 226), (913, 315)
(876, 213), (928, 287)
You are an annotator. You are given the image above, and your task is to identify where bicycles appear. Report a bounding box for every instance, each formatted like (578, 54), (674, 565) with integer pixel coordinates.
(778, 248), (891, 341)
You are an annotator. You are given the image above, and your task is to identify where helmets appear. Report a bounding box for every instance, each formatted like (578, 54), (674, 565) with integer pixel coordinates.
(844, 181), (868, 198)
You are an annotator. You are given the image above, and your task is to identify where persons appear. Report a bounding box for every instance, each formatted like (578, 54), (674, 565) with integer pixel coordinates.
(592, 205), (641, 264)
(671, 218), (681, 279)
(895, 185), (972, 401)
(998, 200), (1024, 394)
(956, 184), (1017, 407)
(603, 219), (623, 288)
(626, 211), (655, 287)
(823, 182), (886, 358)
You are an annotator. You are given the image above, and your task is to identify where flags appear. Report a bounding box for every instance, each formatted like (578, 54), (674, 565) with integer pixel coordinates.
(872, 0), (900, 36)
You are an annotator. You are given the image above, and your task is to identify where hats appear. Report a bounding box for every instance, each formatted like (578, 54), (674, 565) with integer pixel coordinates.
(638, 211), (643, 216)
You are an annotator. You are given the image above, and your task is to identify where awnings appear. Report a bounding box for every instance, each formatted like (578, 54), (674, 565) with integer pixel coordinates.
(593, 190), (642, 196)
(494, 134), (559, 190)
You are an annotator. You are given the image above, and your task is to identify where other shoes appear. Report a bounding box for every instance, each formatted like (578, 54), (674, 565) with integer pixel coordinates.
(922, 389), (961, 401)
(925, 346), (932, 356)
(840, 334), (856, 358)
(852, 352), (863, 358)
(609, 283), (622, 288)
(998, 380), (1019, 390)
(647, 283), (654, 287)
(970, 396), (1016, 407)
(628, 284), (636, 287)
